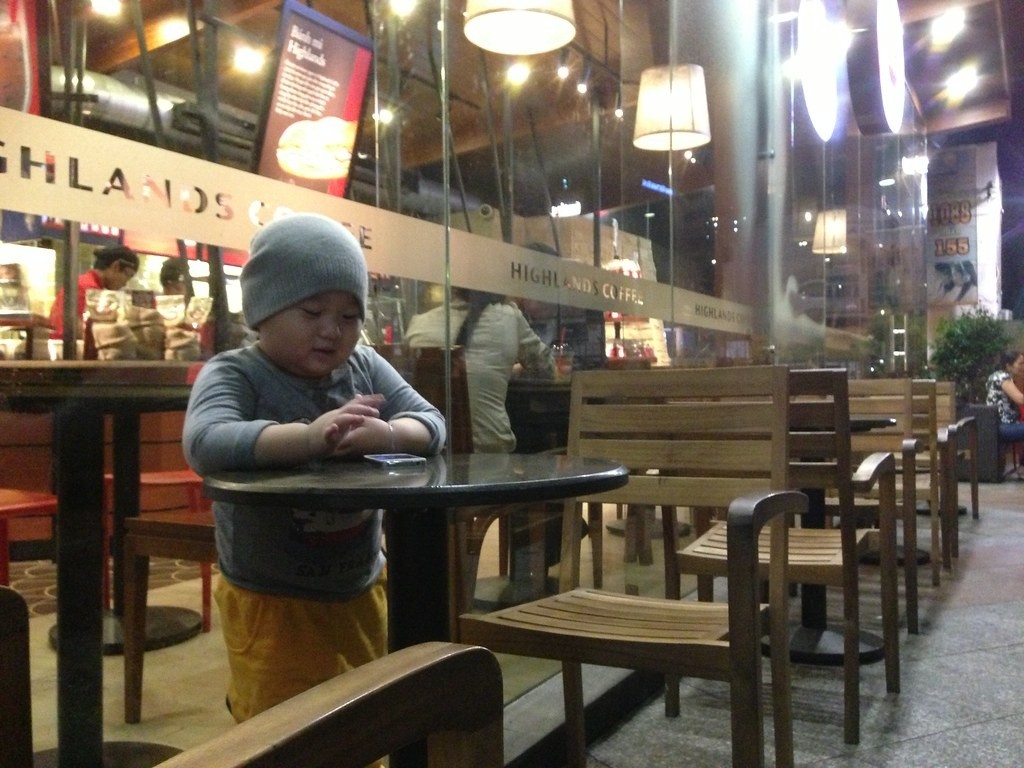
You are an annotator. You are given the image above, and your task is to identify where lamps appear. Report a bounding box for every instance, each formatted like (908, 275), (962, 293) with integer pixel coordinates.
(811, 206), (847, 254)
(463, 0), (578, 56)
(634, 64), (714, 364)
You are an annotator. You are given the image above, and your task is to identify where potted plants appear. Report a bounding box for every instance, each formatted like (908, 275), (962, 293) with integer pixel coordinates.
(930, 306), (1015, 484)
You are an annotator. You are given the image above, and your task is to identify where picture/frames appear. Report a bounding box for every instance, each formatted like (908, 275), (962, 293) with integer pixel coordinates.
(254, 1), (375, 191)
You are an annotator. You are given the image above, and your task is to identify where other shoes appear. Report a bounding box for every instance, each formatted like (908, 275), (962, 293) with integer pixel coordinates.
(1017, 465), (1024, 478)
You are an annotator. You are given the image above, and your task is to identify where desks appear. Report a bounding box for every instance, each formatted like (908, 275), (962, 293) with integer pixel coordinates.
(1, 385), (202, 768)
(196, 452), (637, 767)
(470, 373), (575, 610)
(754, 418), (897, 663)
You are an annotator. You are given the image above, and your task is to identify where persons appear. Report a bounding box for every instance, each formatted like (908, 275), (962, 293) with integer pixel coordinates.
(48, 244), (140, 340)
(985, 351), (1024, 480)
(161, 257), (191, 294)
(405, 241), (607, 567)
(183, 214), (447, 725)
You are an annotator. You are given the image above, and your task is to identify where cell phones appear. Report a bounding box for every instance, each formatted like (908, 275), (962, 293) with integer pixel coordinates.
(363, 453), (427, 468)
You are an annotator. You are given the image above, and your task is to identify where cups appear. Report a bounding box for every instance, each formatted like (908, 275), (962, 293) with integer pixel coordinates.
(551, 344), (575, 385)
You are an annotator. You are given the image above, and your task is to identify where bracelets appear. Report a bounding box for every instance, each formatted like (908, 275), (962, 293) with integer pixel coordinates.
(388, 422), (395, 455)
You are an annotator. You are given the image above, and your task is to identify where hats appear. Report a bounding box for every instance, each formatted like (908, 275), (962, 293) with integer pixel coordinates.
(94, 245), (139, 271)
(241, 215), (368, 329)
(160, 258), (192, 283)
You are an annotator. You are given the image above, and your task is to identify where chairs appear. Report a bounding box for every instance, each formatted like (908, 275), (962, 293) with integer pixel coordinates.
(1, 362), (962, 768)
